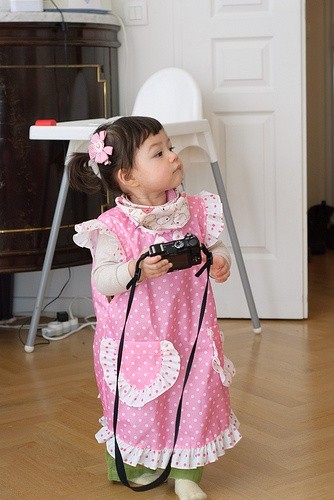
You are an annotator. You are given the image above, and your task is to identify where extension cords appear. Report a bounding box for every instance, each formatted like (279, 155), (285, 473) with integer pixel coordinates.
(41, 317), (79, 337)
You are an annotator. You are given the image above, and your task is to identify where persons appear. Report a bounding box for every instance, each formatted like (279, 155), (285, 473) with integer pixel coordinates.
(66, 117), (243, 499)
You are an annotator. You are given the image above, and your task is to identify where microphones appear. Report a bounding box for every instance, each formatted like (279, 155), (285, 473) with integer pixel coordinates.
(148, 233), (201, 273)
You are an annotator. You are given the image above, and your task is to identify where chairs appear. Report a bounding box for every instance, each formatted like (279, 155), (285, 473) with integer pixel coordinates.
(21, 66), (264, 354)
